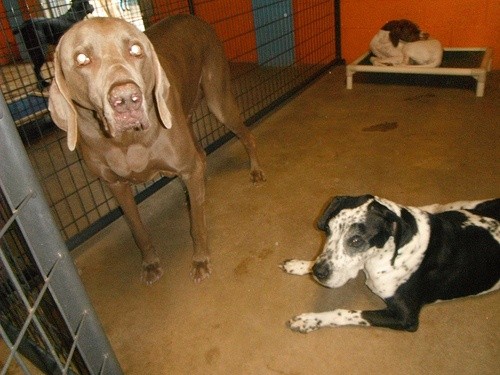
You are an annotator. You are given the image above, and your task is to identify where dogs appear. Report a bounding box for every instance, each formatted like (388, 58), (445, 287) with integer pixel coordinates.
(13, 0), (95, 89)
(46, 14), (266, 286)
(367, 19), (444, 68)
(276, 194), (500, 333)
